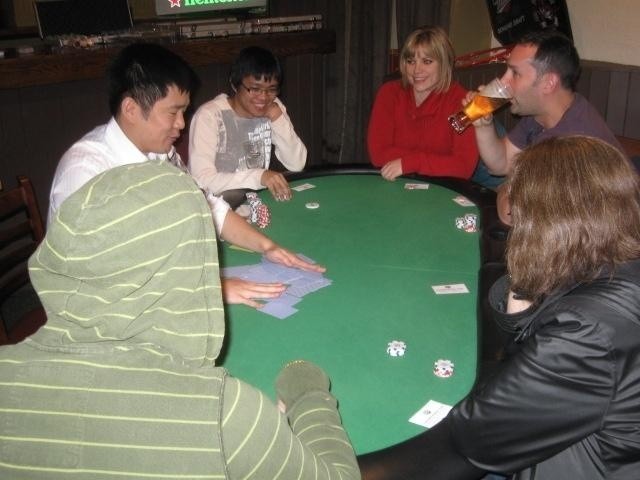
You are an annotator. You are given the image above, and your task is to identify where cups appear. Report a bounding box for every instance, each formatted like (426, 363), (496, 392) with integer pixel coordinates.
(448, 77), (514, 134)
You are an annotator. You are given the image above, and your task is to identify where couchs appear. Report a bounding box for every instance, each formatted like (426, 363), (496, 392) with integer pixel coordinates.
(452, 59), (639, 157)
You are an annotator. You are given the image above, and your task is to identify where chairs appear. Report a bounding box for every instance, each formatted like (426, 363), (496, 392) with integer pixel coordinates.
(1, 174), (46, 345)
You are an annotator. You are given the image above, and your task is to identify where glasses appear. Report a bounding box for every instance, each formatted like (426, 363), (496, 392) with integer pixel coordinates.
(242, 79), (280, 96)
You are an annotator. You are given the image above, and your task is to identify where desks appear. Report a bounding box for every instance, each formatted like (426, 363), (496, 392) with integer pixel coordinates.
(209, 161), (498, 480)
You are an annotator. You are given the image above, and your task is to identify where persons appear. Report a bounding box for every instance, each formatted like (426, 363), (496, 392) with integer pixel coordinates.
(449, 135), (640, 480)
(186, 46), (308, 201)
(45, 42), (326, 308)
(462, 31), (639, 353)
(367, 26), (481, 181)
(0, 157), (361, 480)
